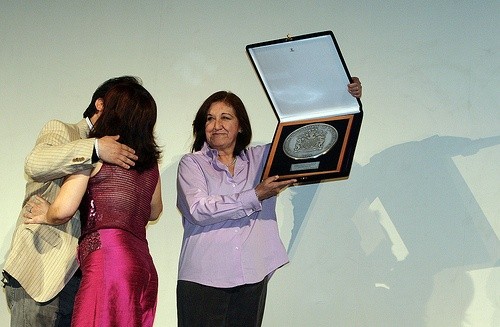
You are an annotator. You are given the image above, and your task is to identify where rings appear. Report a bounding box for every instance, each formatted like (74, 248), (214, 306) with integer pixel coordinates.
(29, 206), (34, 213)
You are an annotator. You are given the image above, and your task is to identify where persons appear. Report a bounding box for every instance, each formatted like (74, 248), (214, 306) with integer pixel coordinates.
(24, 80), (163, 327)
(175, 75), (363, 327)
(0, 74), (142, 326)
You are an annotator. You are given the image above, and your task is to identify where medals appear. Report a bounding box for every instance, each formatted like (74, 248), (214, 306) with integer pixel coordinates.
(281, 122), (339, 160)
